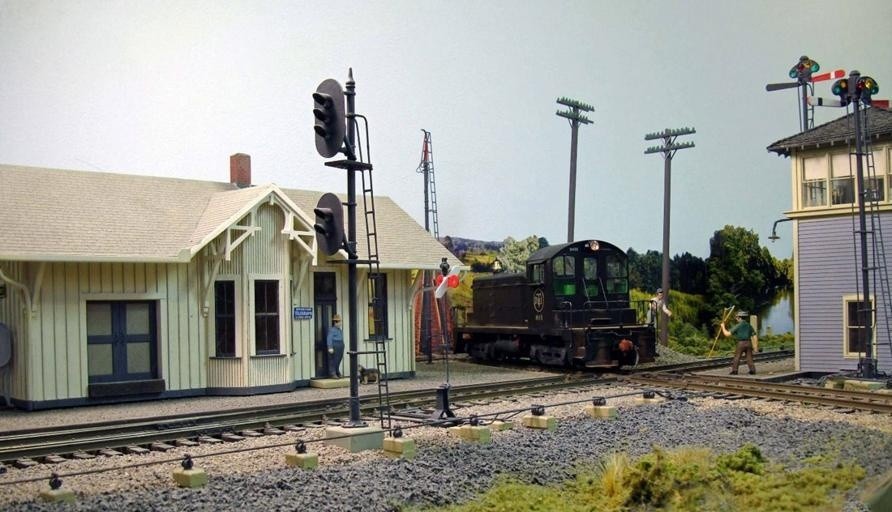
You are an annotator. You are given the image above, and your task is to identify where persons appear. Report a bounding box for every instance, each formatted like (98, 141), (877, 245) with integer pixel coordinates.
(646, 288), (671, 358)
(326, 314), (344, 379)
(721, 311), (760, 374)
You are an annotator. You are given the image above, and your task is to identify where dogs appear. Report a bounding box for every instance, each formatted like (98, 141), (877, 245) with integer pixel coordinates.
(357, 363), (382, 385)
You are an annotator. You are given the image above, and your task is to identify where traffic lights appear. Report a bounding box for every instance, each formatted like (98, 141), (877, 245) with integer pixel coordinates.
(313, 194), (343, 257)
(858, 76), (881, 104)
(311, 78), (345, 158)
(833, 80), (850, 105)
(788, 55), (819, 78)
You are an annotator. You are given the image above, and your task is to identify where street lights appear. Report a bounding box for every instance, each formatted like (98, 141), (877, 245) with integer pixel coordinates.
(643, 125), (695, 346)
(556, 95), (595, 246)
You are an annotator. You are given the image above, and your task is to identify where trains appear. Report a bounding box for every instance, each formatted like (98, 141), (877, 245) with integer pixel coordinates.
(453, 242), (658, 372)
